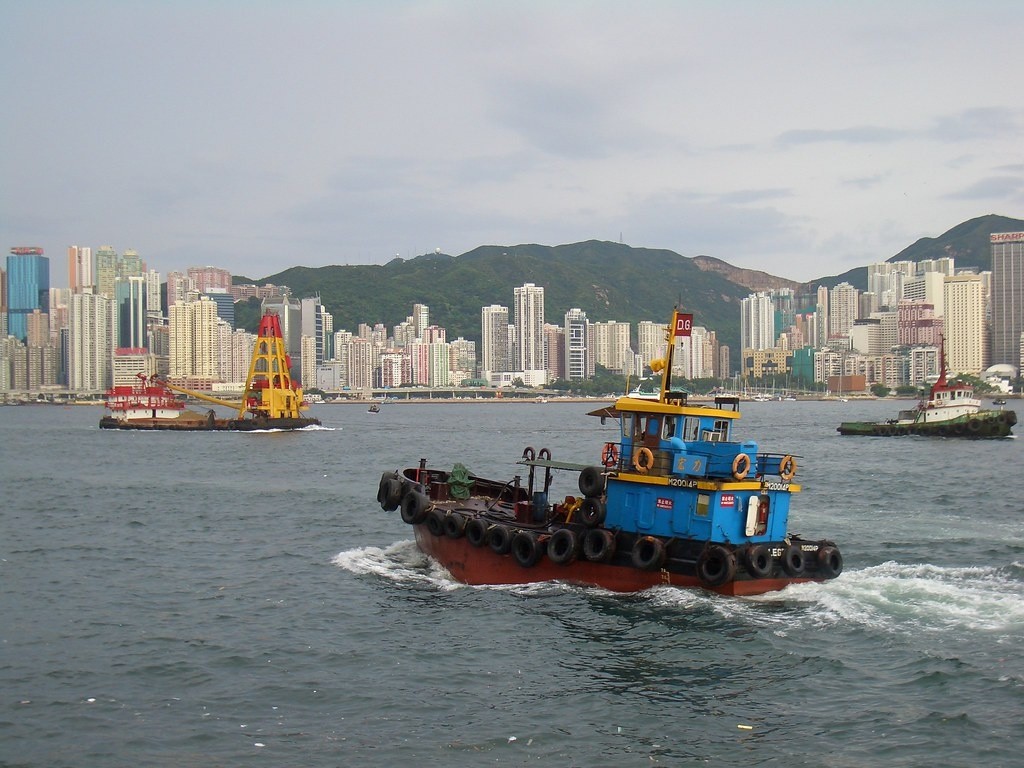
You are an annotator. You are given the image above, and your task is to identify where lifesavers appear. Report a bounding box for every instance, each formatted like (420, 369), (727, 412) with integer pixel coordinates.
(690, 537), (848, 590)
(730, 451), (752, 482)
(871, 410), (1017, 436)
(370, 468), (669, 573)
(600, 442), (619, 469)
(778, 453), (798, 482)
(632, 446), (656, 476)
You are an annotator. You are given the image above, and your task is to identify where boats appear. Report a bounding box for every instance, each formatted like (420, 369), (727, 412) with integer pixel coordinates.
(837, 330), (1018, 439)
(615, 385), (661, 401)
(992, 398), (1006, 405)
(377, 303), (844, 597)
(368, 405), (380, 413)
(97, 308), (321, 430)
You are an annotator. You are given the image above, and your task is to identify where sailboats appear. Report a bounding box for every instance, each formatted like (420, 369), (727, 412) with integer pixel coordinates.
(716, 373), (796, 402)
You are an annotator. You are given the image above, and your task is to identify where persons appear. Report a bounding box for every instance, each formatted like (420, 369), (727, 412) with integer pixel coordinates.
(667, 424), (675, 437)
(693, 427), (698, 440)
